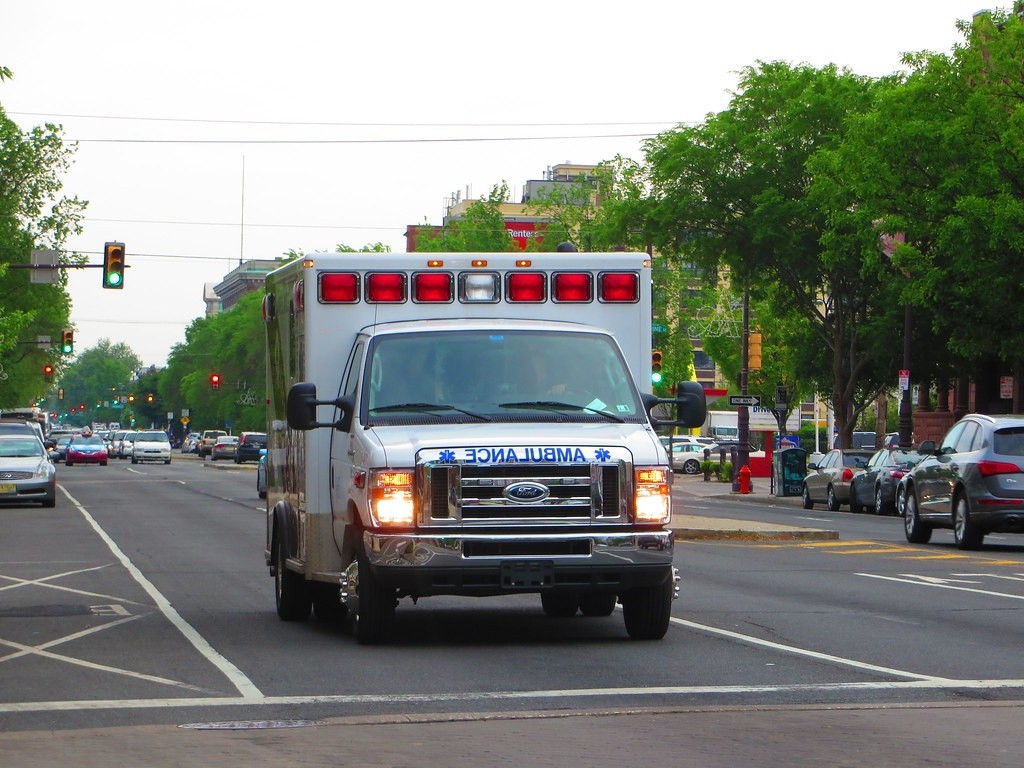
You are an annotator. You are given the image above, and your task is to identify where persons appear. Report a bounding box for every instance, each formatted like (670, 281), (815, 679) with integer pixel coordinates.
(535, 342), (591, 396)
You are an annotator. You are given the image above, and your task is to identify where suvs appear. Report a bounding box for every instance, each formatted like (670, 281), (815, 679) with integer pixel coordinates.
(197, 429), (229, 458)
(902, 413), (1024, 551)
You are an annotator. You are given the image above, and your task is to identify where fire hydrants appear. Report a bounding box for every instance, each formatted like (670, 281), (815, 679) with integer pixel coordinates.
(739, 464), (751, 494)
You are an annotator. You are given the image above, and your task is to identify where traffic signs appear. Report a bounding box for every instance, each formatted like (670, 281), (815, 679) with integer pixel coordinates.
(728, 395), (761, 407)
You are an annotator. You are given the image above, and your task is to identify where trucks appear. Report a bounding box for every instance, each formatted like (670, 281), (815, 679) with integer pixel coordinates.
(700, 410), (740, 440)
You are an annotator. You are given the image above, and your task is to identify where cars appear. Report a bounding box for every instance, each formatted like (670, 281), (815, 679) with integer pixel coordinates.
(180, 432), (202, 455)
(257, 448), (268, 499)
(210, 436), (240, 461)
(64, 425), (110, 466)
(233, 431), (268, 464)
(828, 431), (902, 449)
(801, 448), (881, 512)
(0, 406), (139, 459)
(657, 434), (759, 476)
(130, 428), (174, 464)
(849, 442), (939, 516)
(0, 434), (61, 507)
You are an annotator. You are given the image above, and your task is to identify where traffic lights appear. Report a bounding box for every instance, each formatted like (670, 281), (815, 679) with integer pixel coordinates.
(650, 351), (662, 385)
(103, 242), (125, 289)
(148, 393), (153, 404)
(44, 365), (52, 383)
(62, 328), (74, 354)
(211, 374), (219, 390)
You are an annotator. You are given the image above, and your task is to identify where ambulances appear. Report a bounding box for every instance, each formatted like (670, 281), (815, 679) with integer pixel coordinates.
(267, 248), (709, 645)
